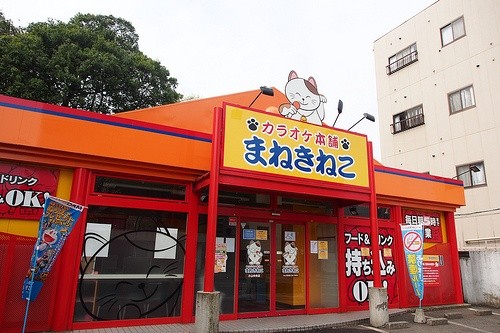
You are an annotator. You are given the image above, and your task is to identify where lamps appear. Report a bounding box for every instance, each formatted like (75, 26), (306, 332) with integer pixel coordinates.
(452, 165), (480, 178)
(248, 86), (274, 108)
(347, 112), (376, 132)
(332, 99), (344, 128)
